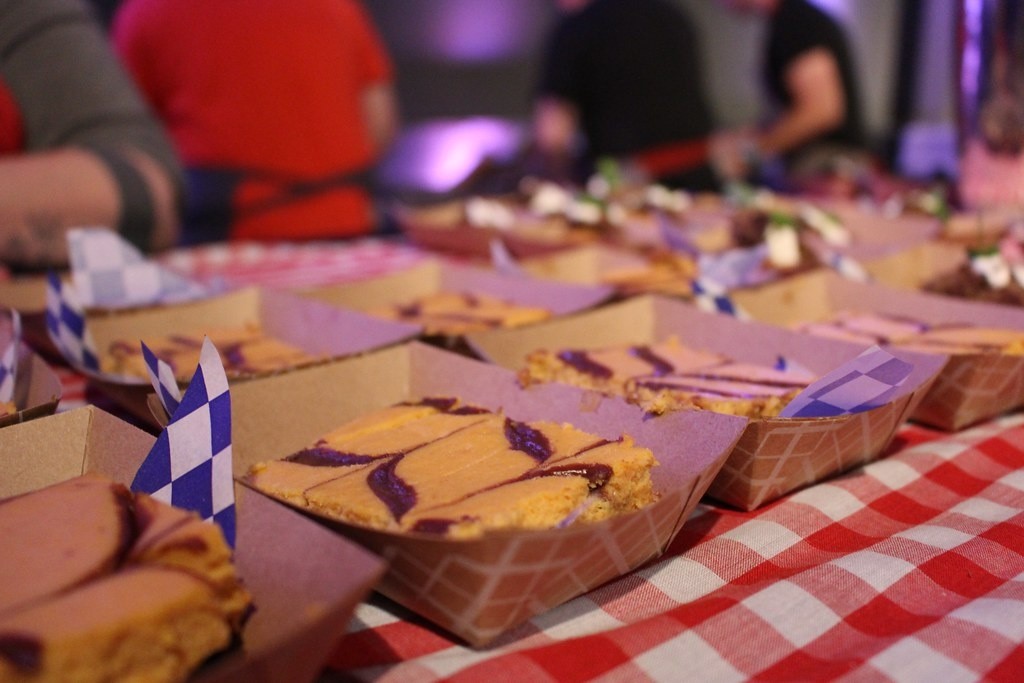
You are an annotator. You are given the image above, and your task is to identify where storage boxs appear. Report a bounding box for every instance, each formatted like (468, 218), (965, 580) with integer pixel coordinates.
(1, 193), (1024, 683)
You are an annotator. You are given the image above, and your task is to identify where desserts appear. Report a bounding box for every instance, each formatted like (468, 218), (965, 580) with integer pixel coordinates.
(0, 205), (1024, 683)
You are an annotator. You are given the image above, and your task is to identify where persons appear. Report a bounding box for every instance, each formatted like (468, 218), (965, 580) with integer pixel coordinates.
(534, 0), (721, 191)
(0, 0), (181, 269)
(111, 0), (399, 240)
(749, 0), (861, 193)
